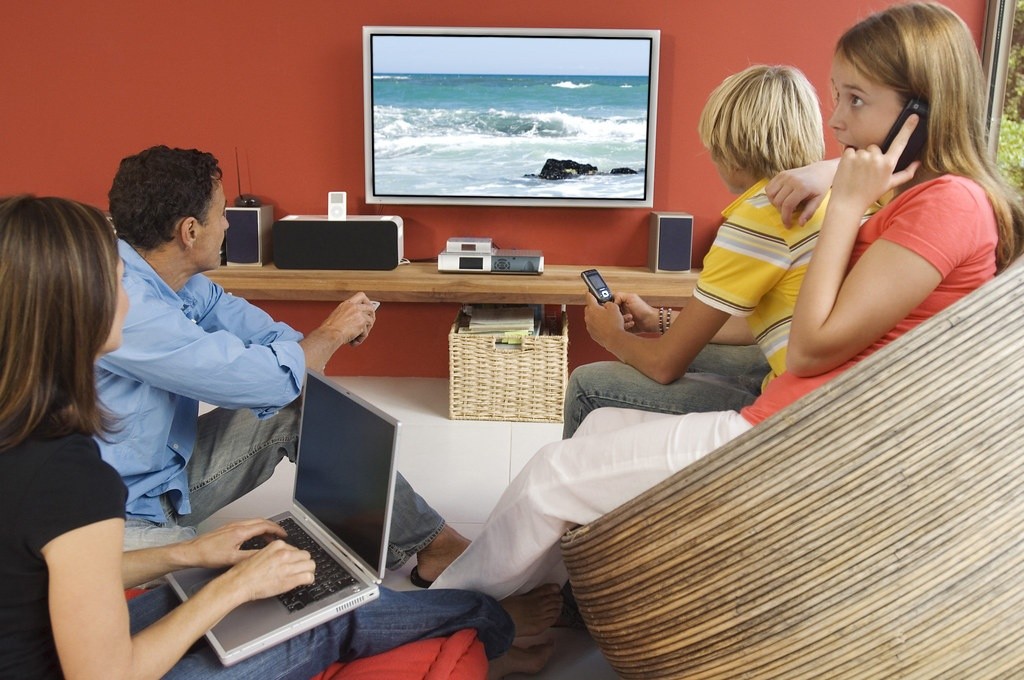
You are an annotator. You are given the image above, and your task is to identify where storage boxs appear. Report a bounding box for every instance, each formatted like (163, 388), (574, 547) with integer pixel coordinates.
(447, 304), (569, 423)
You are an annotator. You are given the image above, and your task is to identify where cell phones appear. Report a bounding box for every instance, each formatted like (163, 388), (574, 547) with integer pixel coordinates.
(879, 98), (930, 175)
(581, 269), (614, 307)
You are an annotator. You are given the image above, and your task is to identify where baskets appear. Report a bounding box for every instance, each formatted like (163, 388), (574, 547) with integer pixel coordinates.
(448, 303), (569, 423)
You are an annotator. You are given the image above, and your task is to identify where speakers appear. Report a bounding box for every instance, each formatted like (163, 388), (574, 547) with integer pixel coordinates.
(225, 205), (273, 266)
(272, 215), (404, 272)
(646, 211), (694, 274)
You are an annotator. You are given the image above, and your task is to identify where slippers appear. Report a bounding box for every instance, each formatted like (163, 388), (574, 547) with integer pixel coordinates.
(410, 564), (434, 589)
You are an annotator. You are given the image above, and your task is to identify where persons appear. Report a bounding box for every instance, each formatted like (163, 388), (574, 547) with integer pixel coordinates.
(558, 59), (886, 440)
(1, 192), (566, 679)
(427, 0), (1024, 602)
(83, 142), (379, 532)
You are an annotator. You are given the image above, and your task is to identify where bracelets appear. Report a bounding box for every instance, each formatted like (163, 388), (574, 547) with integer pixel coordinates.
(658, 304), (673, 337)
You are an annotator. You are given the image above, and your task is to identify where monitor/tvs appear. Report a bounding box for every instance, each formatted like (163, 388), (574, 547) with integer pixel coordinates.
(361, 25), (661, 207)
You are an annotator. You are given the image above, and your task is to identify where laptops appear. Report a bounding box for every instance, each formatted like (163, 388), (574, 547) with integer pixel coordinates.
(165, 367), (402, 667)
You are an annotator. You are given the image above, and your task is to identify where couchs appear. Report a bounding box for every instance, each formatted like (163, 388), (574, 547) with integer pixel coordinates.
(556, 252), (1024, 680)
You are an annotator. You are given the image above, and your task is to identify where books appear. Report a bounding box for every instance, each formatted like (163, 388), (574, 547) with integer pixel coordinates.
(456, 303), (565, 350)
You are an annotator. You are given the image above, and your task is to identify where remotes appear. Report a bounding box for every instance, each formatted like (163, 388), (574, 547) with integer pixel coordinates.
(370, 301), (380, 310)
